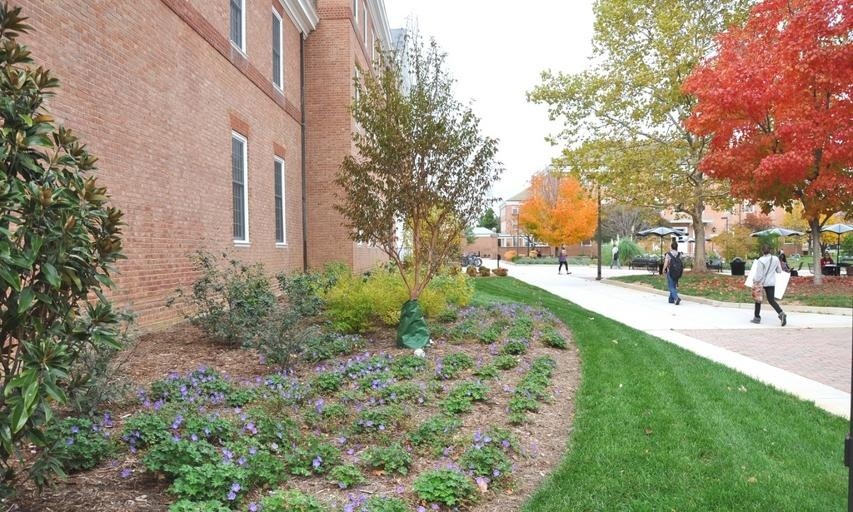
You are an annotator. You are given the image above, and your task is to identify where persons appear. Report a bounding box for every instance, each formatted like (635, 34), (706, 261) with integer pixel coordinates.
(749, 244), (787, 327)
(661, 240), (684, 305)
(609, 243), (621, 269)
(778, 248), (790, 273)
(557, 243), (572, 275)
(819, 254), (835, 276)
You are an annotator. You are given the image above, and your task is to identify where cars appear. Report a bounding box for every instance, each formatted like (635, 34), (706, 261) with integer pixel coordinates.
(628, 252), (725, 270)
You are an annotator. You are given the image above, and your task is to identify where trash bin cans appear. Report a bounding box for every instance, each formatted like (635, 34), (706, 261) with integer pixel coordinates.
(730, 257), (746, 275)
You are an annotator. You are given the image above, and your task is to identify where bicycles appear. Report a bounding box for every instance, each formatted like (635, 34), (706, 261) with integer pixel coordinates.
(459, 253), (482, 267)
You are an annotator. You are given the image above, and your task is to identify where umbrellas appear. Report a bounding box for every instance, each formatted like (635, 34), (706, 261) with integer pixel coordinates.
(749, 227), (807, 251)
(806, 223), (853, 264)
(635, 226), (686, 264)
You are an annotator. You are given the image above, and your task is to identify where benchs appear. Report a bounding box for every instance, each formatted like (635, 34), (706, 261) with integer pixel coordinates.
(704, 262), (723, 272)
(627, 257), (664, 269)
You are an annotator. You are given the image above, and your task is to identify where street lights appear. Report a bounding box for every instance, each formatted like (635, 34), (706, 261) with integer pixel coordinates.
(720, 216), (728, 231)
(491, 227), (496, 234)
(805, 227), (812, 256)
(711, 226), (716, 252)
(511, 213), (518, 257)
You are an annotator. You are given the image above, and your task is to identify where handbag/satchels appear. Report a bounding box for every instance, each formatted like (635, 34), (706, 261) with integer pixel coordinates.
(752, 281), (763, 303)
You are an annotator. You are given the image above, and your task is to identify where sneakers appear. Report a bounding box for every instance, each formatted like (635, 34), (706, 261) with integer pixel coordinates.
(669, 297), (681, 305)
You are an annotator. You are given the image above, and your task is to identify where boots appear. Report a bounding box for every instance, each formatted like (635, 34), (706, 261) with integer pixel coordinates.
(750, 316), (760, 324)
(778, 312), (786, 326)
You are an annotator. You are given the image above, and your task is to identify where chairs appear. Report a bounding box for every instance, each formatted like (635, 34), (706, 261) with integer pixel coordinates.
(809, 262), (816, 275)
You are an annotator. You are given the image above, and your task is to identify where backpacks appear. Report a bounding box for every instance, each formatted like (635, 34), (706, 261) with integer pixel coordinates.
(667, 251), (682, 281)
